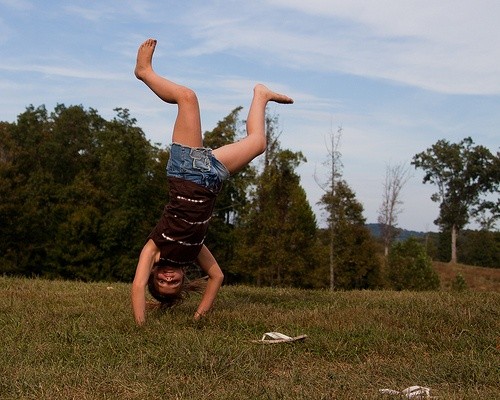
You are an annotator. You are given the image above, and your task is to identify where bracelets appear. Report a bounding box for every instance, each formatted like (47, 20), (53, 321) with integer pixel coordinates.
(196, 310), (201, 316)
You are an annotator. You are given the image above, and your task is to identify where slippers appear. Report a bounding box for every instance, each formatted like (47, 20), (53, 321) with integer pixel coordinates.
(379, 386), (439, 399)
(249, 331), (307, 344)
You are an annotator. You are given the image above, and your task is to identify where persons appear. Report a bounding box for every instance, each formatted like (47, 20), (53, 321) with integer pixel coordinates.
(131, 39), (293, 326)
